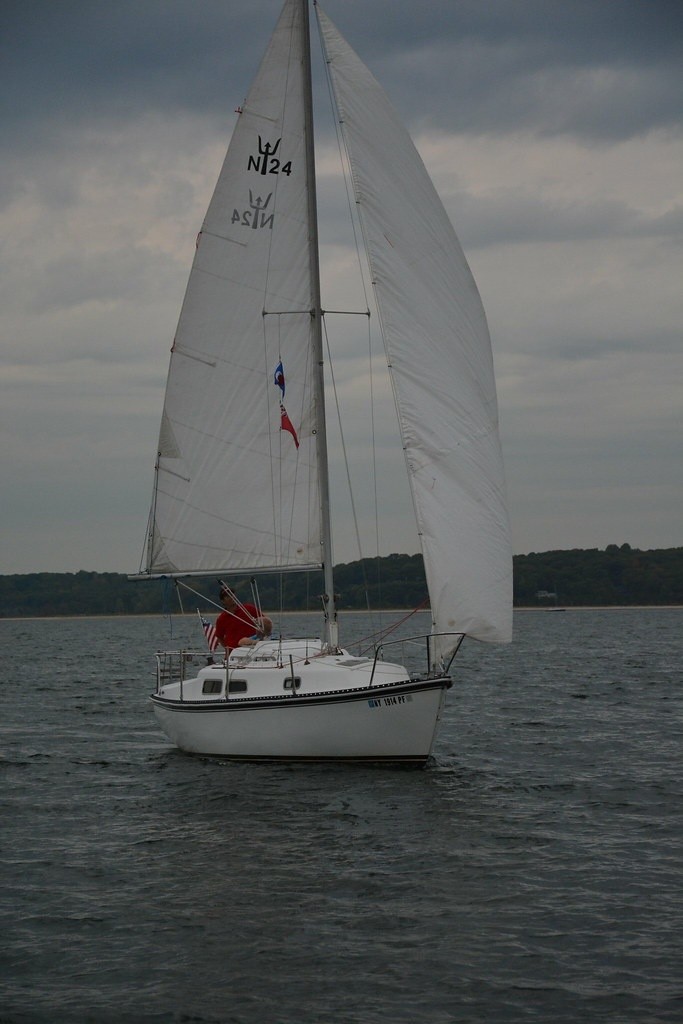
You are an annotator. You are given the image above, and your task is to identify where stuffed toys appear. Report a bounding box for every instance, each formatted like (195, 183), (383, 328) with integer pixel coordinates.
(238, 617), (272, 646)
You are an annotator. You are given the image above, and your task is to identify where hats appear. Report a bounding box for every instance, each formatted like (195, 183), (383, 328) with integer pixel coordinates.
(220, 587), (235, 599)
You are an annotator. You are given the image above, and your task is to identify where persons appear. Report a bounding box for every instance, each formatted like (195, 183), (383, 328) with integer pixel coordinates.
(215, 588), (265, 660)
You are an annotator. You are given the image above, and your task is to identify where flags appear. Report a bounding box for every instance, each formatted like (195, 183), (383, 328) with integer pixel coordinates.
(273, 361), (285, 401)
(200, 616), (218, 652)
(280, 404), (299, 449)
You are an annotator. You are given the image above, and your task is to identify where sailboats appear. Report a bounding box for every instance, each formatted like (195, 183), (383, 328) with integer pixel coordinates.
(126, 0), (512, 766)
